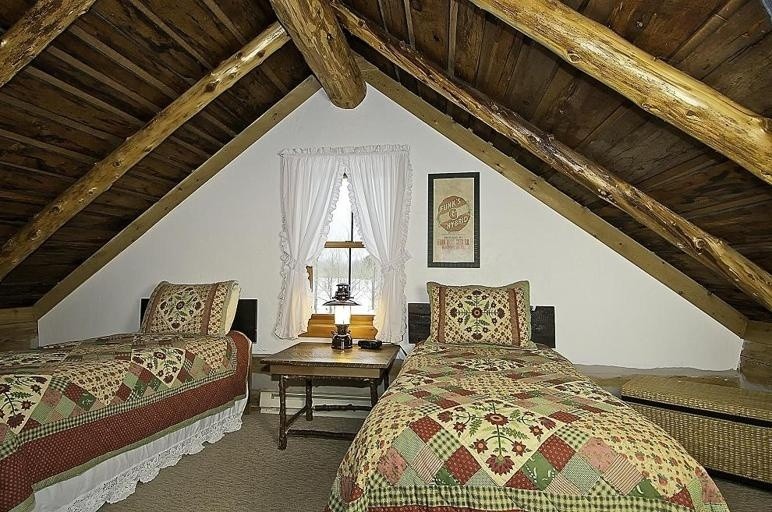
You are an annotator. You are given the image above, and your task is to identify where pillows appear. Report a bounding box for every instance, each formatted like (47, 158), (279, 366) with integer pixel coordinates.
(426, 280), (537, 350)
(134, 280), (240, 335)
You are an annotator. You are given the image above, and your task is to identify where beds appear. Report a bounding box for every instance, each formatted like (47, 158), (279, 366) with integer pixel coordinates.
(358, 305), (735, 512)
(1, 300), (256, 512)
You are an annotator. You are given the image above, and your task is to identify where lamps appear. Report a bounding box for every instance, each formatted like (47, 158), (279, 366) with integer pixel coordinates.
(322, 284), (360, 350)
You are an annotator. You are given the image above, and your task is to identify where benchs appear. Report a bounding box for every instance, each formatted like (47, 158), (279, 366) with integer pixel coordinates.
(619, 369), (771, 485)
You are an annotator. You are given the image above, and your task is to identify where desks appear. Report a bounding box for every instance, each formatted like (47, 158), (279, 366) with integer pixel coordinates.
(259, 342), (400, 451)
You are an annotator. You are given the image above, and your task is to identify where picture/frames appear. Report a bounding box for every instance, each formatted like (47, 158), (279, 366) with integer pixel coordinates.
(427, 172), (480, 270)
